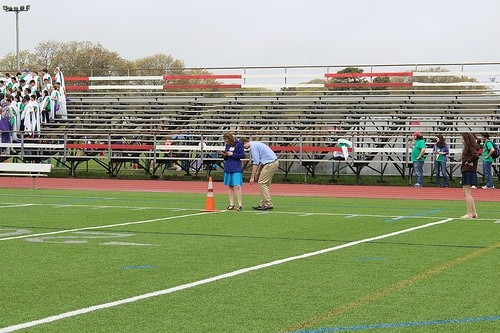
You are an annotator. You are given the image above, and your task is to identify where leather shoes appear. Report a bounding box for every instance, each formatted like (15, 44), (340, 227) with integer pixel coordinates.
(258, 205), (273, 210)
(252, 205), (264, 210)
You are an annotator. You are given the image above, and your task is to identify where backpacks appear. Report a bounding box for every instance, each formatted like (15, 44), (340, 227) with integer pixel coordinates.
(485, 141), (500, 160)
(478, 145), (483, 156)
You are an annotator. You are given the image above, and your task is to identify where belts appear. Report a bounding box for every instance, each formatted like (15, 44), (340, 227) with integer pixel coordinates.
(263, 159), (277, 166)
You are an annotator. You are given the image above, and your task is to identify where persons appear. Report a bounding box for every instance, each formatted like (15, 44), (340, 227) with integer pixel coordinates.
(460, 132), (480, 219)
(471, 138), (482, 189)
(433, 135), (449, 188)
(220, 133), (246, 211)
(409, 131), (426, 187)
(239, 137), (279, 210)
(481, 132), (495, 190)
(0, 67), (67, 155)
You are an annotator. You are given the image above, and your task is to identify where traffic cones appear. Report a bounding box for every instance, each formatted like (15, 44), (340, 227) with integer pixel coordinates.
(199, 177), (219, 213)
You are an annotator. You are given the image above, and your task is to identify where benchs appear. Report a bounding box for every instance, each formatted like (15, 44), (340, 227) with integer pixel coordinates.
(0, 96), (500, 186)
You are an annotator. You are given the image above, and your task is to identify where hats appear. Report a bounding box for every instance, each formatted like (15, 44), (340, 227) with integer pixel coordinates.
(413, 131), (422, 136)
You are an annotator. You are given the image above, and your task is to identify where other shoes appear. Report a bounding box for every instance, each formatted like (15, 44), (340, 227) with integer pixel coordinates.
(227, 204), (235, 210)
(236, 205), (242, 211)
(472, 214), (478, 218)
(461, 213), (473, 219)
